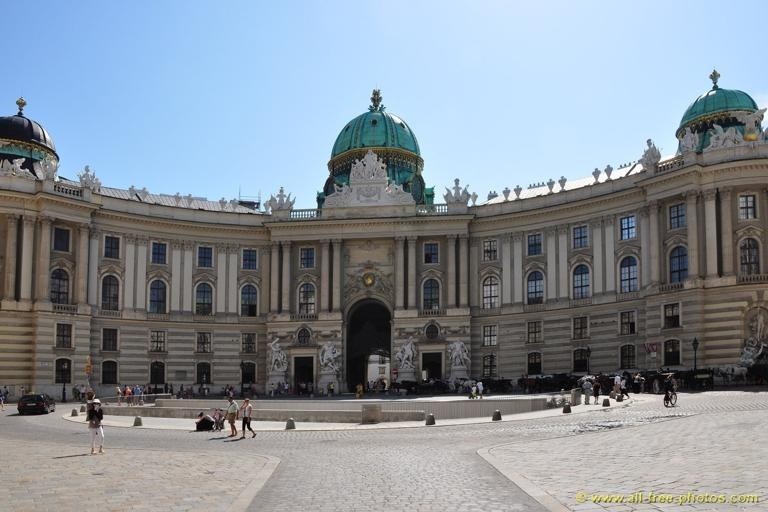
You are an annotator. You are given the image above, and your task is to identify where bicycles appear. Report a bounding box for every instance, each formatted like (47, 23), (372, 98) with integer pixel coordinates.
(662, 385), (678, 407)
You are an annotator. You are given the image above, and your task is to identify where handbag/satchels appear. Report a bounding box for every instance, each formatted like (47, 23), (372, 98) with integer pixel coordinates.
(87, 416), (100, 428)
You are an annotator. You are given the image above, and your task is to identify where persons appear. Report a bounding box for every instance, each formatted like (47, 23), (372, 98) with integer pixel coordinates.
(452, 178), (462, 199)
(757, 310), (764, 341)
(0, 367), (676, 438)
(276, 187), (286, 205)
(328, 150), (406, 198)
(319, 341), (336, 369)
(86, 399), (104, 454)
(643, 137), (656, 160)
(271, 337), (282, 371)
(82, 165), (95, 188)
(451, 337), (468, 367)
(398, 339), (413, 368)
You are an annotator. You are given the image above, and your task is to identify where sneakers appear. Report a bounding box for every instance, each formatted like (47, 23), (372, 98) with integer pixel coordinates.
(91, 451), (104, 454)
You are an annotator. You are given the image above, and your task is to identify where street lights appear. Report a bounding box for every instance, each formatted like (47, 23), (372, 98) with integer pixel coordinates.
(60, 359), (68, 402)
(691, 337), (700, 370)
(240, 359), (245, 397)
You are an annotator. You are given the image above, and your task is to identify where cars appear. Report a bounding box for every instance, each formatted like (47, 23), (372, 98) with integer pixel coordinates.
(689, 368), (713, 388)
(402, 371), (678, 395)
(18, 393), (56, 414)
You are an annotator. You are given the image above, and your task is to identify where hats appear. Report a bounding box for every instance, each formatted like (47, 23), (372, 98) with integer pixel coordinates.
(91, 399), (101, 407)
